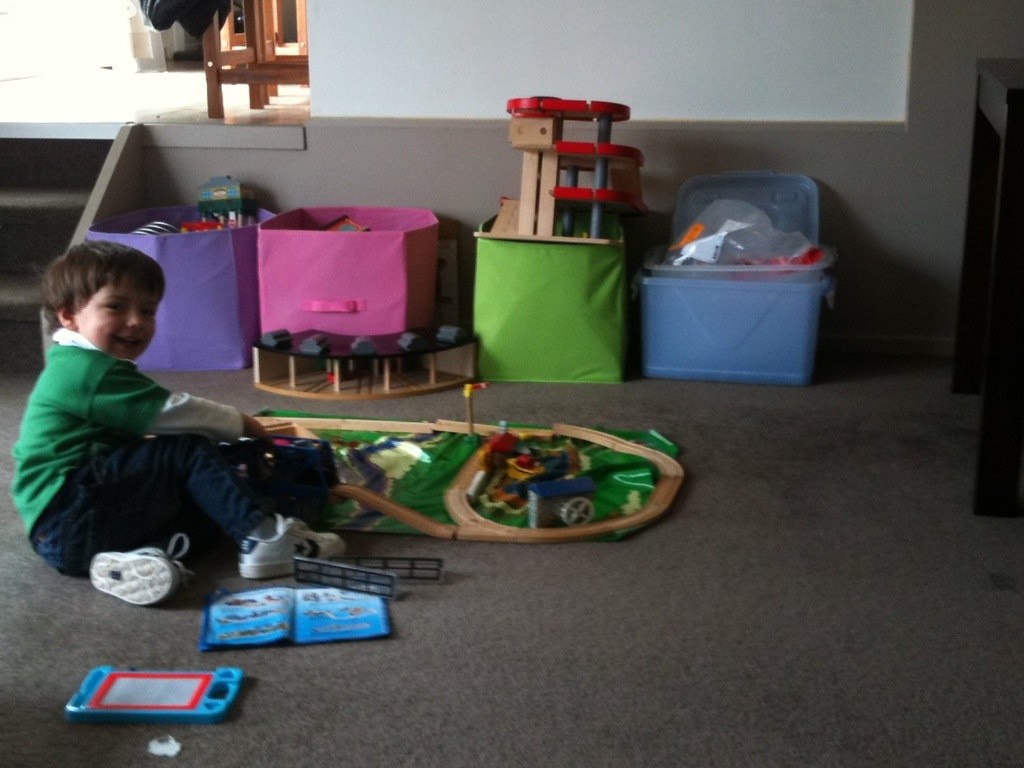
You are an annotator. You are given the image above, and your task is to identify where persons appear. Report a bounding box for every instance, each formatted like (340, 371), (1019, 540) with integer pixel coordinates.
(11, 239), (346, 606)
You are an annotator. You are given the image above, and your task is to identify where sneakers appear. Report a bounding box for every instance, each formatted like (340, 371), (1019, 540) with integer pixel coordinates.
(238, 512), (346, 578)
(89, 534), (195, 606)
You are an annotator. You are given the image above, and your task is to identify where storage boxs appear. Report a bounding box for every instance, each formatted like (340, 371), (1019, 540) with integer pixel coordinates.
(642, 171), (836, 383)
(257, 208), (441, 337)
(474, 212), (634, 385)
(89, 206), (276, 372)
